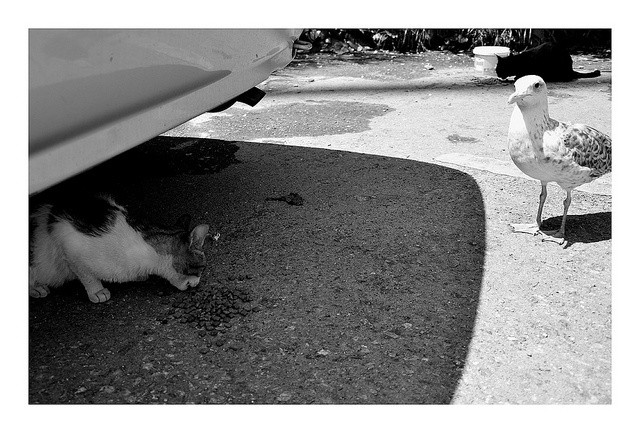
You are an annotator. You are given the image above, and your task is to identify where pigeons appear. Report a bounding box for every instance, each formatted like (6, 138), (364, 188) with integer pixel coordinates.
(508, 74), (611, 247)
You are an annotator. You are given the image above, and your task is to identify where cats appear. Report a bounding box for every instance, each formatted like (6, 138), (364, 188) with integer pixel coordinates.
(28, 182), (210, 302)
